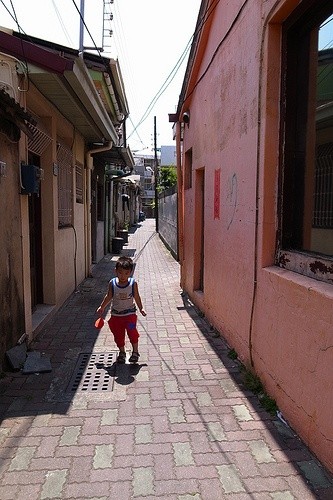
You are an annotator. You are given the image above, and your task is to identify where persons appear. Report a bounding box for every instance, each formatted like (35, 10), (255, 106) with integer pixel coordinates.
(95, 255), (148, 364)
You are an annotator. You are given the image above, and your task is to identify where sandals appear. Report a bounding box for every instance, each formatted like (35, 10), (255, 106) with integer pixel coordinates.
(117, 352), (126, 362)
(129, 352), (140, 362)
